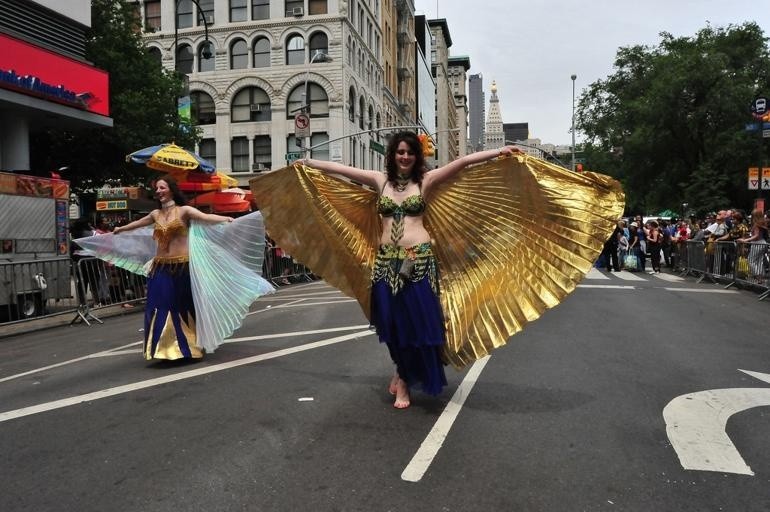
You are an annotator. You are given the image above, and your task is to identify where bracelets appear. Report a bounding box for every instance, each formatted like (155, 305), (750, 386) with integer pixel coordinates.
(498, 148), (503, 156)
(304, 158), (307, 166)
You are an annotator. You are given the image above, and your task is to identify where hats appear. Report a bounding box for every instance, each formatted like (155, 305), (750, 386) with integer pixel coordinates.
(629, 222), (638, 227)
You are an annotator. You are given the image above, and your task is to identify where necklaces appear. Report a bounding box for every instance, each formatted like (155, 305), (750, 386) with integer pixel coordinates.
(392, 171), (410, 192)
(161, 200), (175, 221)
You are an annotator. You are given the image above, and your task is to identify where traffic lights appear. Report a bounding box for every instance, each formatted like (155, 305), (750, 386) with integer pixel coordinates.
(419, 132), (434, 158)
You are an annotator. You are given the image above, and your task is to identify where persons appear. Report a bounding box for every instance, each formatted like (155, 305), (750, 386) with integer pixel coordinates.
(594, 208), (770, 284)
(113, 174), (237, 364)
(262, 231), (321, 285)
(291, 131), (524, 409)
(69, 212), (148, 308)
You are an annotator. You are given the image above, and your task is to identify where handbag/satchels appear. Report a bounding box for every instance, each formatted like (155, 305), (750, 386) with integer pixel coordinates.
(623, 248), (637, 269)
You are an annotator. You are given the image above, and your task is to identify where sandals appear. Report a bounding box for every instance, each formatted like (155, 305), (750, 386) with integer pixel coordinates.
(280, 272), (291, 285)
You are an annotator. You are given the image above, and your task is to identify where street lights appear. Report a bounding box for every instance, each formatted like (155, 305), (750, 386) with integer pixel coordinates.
(300, 51), (334, 160)
(174, 1), (212, 148)
(570, 70), (578, 170)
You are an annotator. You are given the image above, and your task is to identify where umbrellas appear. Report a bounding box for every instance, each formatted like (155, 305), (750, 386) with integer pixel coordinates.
(210, 200), (251, 213)
(186, 187), (258, 209)
(159, 168), (239, 192)
(126, 141), (217, 178)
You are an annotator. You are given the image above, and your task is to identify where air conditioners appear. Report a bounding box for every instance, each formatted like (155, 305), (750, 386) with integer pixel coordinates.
(252, 164), (264, 171)
(203, 16), (214, 23)
(251, 104), (262, 112)
(293, 7), (303, 16)
